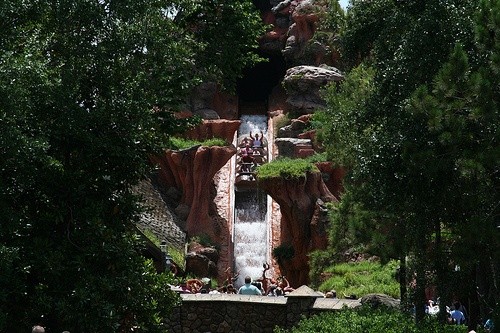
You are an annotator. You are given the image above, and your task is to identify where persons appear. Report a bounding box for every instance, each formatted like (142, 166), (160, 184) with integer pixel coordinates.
(177, 275), (359, 303)
(238, 130), (265, 181)
(425, 297), (500, 333)
